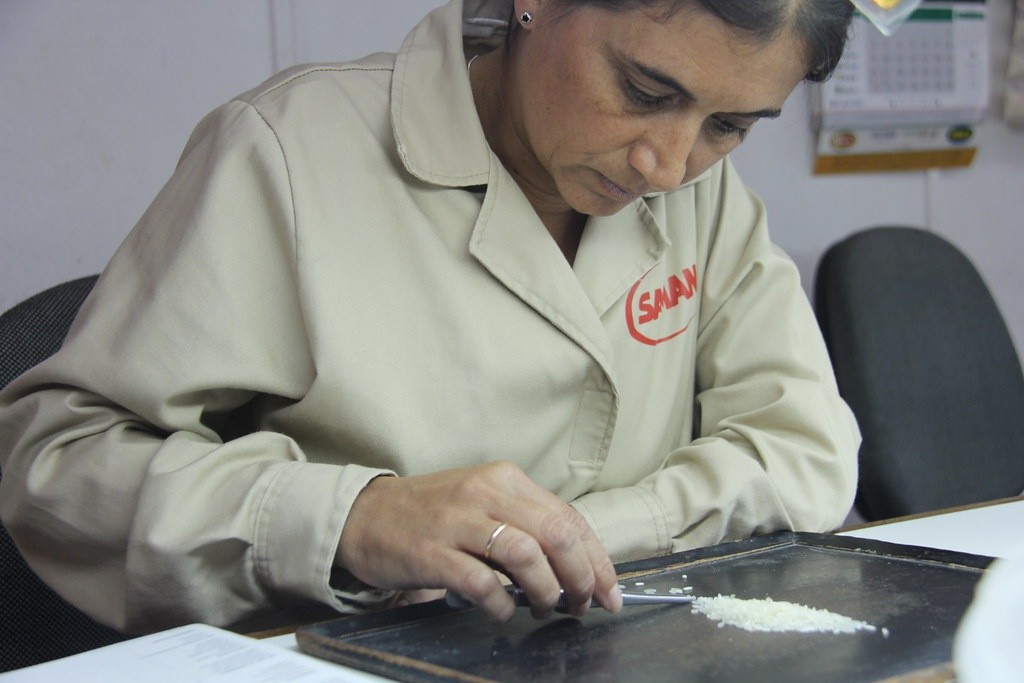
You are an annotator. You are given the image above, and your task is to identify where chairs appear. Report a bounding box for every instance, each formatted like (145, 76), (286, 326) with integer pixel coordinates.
(810, 225), (1024, 522)
(0, 275), (256, 678)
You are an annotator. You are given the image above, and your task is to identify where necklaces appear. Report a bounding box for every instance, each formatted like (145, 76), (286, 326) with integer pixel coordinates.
(467, 54), (479, 75)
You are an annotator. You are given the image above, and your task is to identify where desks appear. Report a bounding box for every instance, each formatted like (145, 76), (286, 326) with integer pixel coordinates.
(270, 494), (1024, 683)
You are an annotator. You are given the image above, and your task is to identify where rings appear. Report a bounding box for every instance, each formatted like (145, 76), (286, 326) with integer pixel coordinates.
(483, 522), (508, 559)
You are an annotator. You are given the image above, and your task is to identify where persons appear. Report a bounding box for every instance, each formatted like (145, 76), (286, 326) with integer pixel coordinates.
(0, 0), (864, 641)
